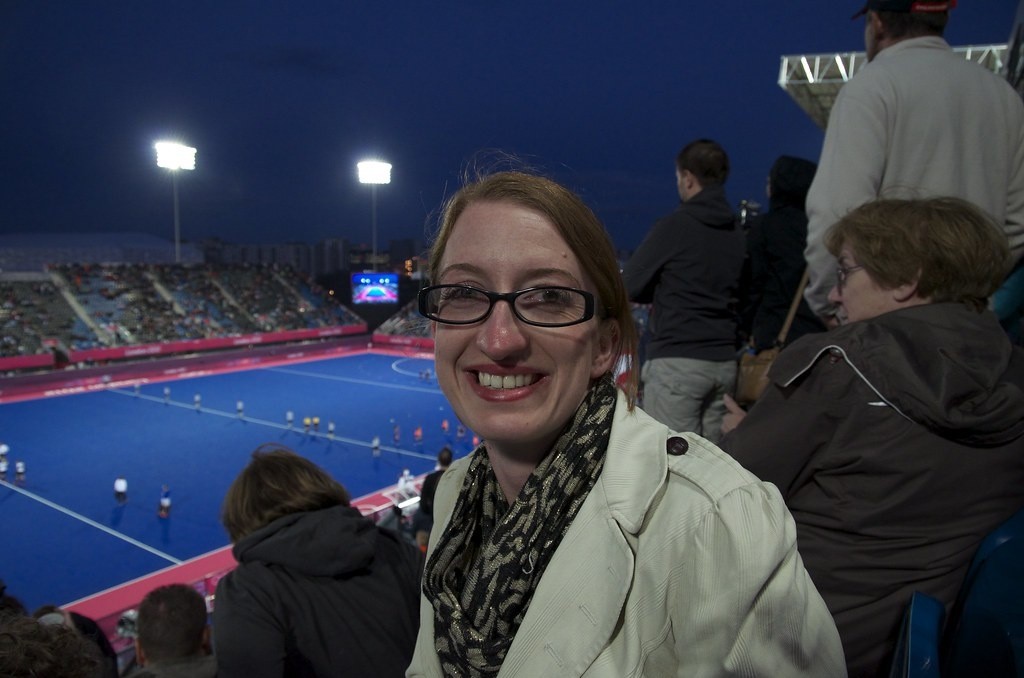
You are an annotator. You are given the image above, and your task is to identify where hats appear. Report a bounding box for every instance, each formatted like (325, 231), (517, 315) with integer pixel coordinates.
(850, 0), (957, 21)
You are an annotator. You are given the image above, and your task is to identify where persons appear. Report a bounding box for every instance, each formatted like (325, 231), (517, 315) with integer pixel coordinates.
(717, 0), (1022, 667)
(402, 171), (849, 677)
(0, 371), (479, 678)
(624, 134), (737, 439)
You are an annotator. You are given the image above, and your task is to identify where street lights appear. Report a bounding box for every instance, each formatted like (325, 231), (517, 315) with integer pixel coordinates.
(357, 161), (392, 270)
(154, 142), (197, 260)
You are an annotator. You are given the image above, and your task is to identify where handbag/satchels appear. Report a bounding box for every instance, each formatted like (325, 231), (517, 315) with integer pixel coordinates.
(736, 337), (785, 402)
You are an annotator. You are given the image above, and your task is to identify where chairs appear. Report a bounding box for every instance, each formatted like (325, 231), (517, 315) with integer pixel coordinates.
(0, 260), (357, 358)
(949, 517), (1024, 676)
(887, 590), (940, 677)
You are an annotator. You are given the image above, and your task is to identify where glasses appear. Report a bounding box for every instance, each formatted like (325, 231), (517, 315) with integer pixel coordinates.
(835, 259), (920, 287)
(417, 284), (606, 328)
